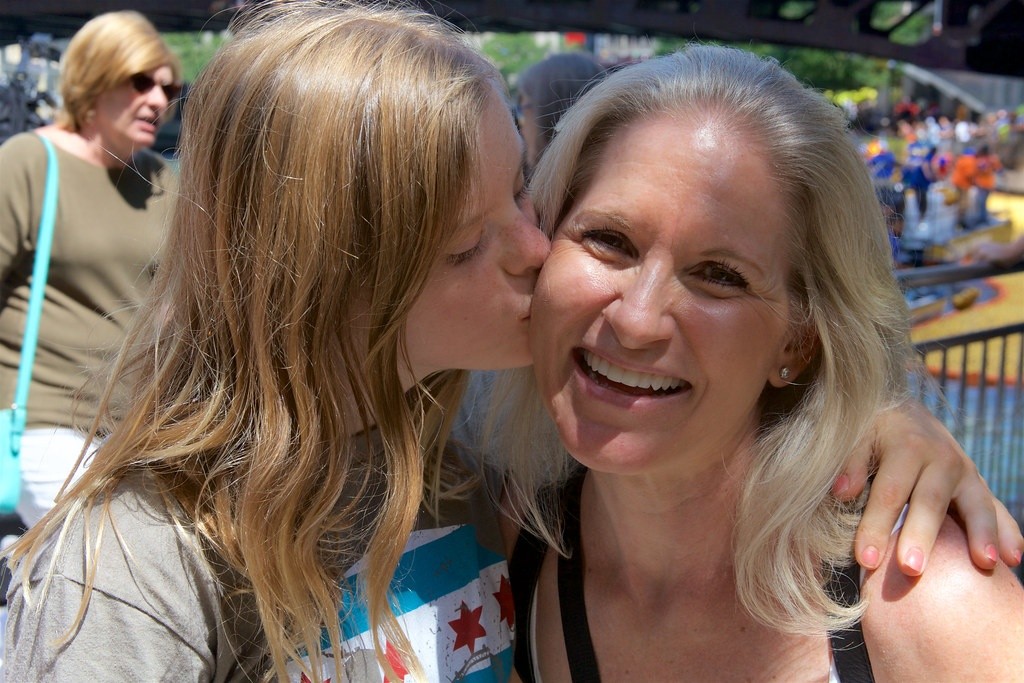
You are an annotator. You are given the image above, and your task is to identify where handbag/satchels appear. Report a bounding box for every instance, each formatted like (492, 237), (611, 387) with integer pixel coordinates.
(0, 408), (22, 515)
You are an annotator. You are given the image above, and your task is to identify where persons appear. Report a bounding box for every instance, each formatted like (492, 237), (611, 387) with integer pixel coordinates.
(425, 51), (610, 563)
(0, 9), (186, 538)
(480, 42), (1024, 683)
(854, 111), (1000, 255)
(0, 5), (1022, 679)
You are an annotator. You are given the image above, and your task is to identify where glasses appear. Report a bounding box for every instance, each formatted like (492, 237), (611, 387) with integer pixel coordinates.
(129, 72), (179, 101)
